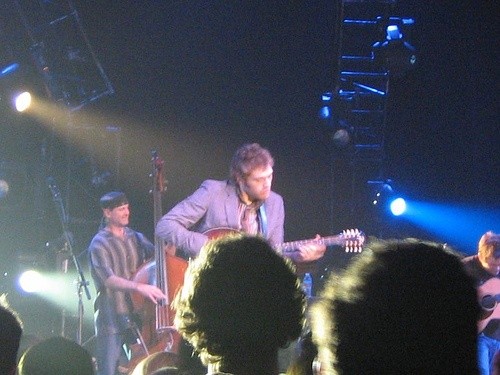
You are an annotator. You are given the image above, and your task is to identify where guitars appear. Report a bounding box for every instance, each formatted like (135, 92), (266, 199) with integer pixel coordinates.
(478, 277), (500, 335)
(188, 227), (367, 257)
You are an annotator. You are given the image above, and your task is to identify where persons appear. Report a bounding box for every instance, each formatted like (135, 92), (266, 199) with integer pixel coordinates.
(88, 191), (177, 375)
(310, 238), (485, 375)
(170, 231), (308, 375)
(17, 336), (95, 375)
(156, 145), (326, 262)
(458, 230), (500, 375)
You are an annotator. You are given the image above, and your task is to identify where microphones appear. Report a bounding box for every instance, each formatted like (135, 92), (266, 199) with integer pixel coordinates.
(46, 176), (61, 200)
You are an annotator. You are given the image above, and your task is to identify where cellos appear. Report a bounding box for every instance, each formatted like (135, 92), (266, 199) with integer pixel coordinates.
(129, 150), (190, 375)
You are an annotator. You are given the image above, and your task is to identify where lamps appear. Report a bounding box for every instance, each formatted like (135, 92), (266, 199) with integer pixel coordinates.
(372, 182), (407, 217)
(369, 17), (419, 65)
